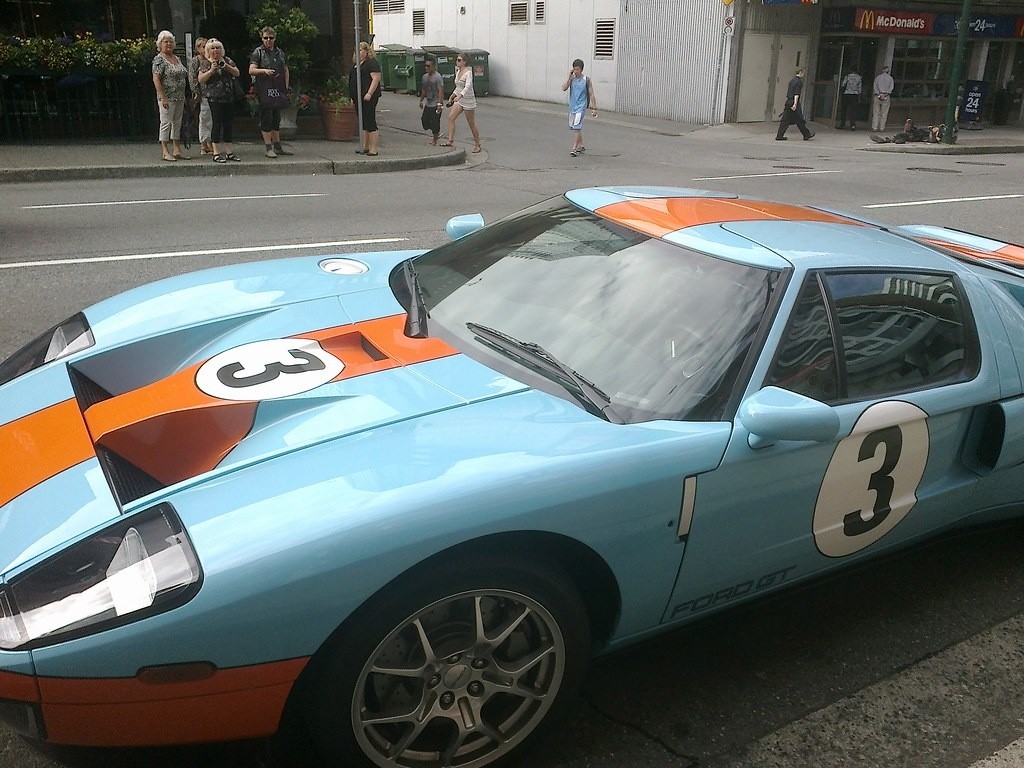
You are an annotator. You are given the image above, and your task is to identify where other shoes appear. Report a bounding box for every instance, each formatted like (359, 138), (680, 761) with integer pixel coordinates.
(273, 149), (294, 156)
(199, 143), (214, 155)
(265, 149), (278, 158)
(803, 132), (815, 140)
(775, 137), (787, 140)
(577, 145), (586, 152)
(869, 134), (884, 143)
(174, 153), (191, 160)
(437, 132), (444, 139)
(354, 149), (378, 156)
(836, 123), (856, 131)
(161, 153), (177, 161)
(570, 148), (578, 158)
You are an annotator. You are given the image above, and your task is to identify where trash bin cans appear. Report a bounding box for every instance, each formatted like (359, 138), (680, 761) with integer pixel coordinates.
(374, 43), (491, 99)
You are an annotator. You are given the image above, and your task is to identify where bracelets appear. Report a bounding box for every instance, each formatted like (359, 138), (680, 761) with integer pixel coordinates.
(592, 108), (596, 110)
(437, 103), (442, 106)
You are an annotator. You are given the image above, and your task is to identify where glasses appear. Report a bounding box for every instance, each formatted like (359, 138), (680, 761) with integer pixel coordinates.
(425, 64), (430, 66)
(455, 58), (464, 62)
(263, 37), (274, 39)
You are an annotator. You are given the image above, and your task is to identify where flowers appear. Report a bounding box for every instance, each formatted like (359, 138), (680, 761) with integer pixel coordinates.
(246, 83), (311, 111)
(0, 32), (158, 80)
(317, 76), (357, 119)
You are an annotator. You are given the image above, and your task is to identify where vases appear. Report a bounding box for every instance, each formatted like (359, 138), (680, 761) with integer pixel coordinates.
(258, 108), (299, 140)
(317, 101), (356, 141)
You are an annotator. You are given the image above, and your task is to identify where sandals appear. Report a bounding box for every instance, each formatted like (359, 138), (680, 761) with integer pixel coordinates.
(225, 153), (241, 162)
(472, 145), (481, 153)
(440, 141), (452, 147)
(213, 155), (226, 163)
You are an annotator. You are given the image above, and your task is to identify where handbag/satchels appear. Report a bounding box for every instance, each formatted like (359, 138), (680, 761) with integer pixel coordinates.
(180, 105), (198, 139)
(229, 77), (244, 109)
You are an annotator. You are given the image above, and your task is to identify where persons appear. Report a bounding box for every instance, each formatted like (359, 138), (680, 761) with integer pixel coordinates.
(189, 38), (242, 163)
(775, 67), (815, 140)
(349, 42), (382, 156)
(871, 66), (894, 132)
(835, 68), (862, 131)
(152, 30), (191, 161)
(440, 55), (482, 153)
(248, 27), (294, 158)
(993, 75), (1017, 124)
(419, 57), (444, 146)
(563, 58), (597, 156)
(870, 118), (958, 144)
